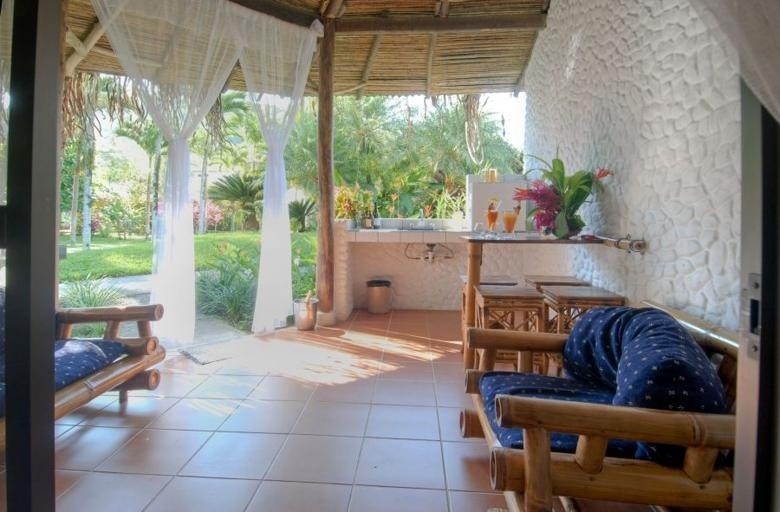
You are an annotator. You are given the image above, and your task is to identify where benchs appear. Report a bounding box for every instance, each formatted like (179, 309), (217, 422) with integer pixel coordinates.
(459, 299), (739, 512)
(0, 287), (165, 442)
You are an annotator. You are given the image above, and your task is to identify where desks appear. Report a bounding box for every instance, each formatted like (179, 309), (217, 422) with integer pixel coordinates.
(460, 233), (605, 368)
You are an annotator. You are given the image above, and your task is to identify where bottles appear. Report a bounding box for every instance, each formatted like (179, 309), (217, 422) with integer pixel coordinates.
(304, 290), (312, 304)
(481, 169), (497, 184)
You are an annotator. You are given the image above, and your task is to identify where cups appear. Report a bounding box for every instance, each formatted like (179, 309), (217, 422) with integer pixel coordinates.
(502, 210), (517, 235)
(487, 209), (498, 232)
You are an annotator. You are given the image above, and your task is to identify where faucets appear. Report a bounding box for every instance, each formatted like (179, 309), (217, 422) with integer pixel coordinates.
(418, 208), (425, 224)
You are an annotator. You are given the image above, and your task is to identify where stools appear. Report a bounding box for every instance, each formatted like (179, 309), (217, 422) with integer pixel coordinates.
(473, 285), (547, 376)
(523, 275), (590, 331)
(540, 285), (625, 377)
(459, 275), (519, 353)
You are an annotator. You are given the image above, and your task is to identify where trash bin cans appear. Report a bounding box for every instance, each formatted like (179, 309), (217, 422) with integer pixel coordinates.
(366, 280), (391, 314)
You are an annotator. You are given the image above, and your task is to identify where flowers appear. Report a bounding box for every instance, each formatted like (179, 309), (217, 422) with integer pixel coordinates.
(512, 144), (612, 235)
(335, 186), (374, 219)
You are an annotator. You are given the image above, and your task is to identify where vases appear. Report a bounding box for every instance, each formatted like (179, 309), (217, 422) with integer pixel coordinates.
(349, 217), (364, 229)
(551, 214), (584, 239)
